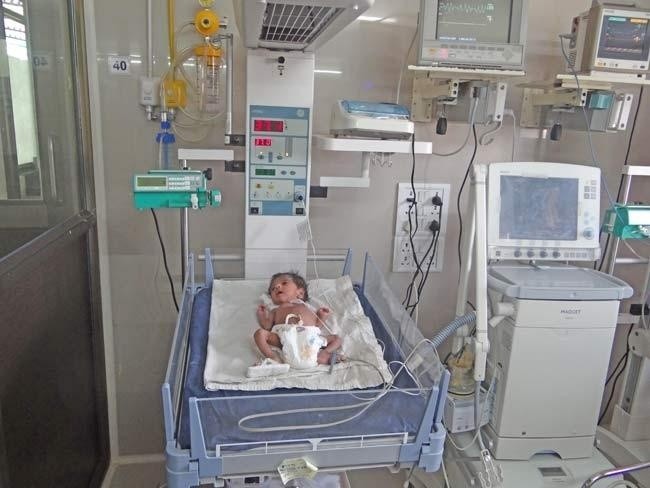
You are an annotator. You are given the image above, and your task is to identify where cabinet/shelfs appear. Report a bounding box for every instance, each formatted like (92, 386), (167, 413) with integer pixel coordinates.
(487, 277), (621, 460)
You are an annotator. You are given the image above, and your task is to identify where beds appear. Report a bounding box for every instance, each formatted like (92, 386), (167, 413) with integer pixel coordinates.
(158, 247), (450, 488)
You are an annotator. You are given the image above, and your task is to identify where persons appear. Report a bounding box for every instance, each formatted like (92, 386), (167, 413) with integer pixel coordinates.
(253, 270), (343, 365)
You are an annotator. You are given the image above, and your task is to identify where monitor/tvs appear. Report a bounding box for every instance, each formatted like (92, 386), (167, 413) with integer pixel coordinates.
(489, 163), (601, 260)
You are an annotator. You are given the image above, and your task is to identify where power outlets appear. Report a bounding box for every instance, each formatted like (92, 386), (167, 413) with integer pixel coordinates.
(391, 182), (451, 273)
